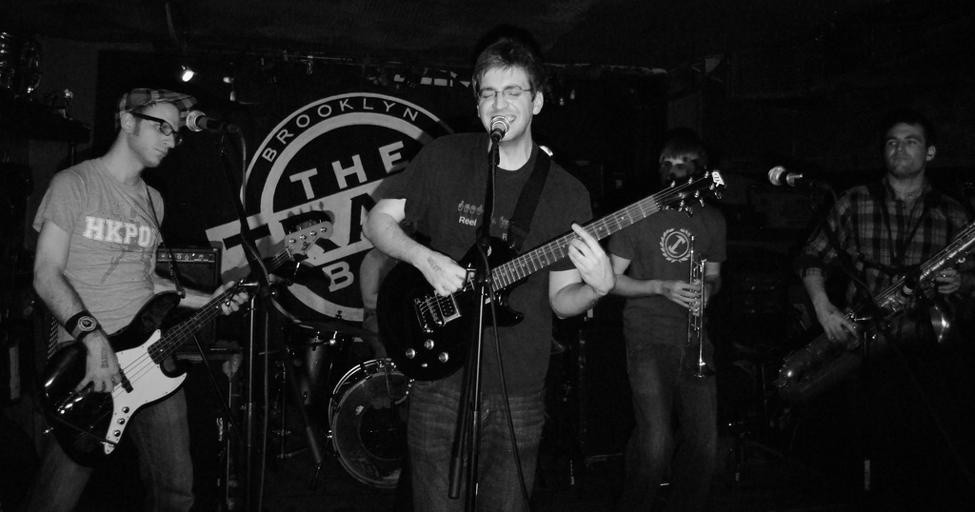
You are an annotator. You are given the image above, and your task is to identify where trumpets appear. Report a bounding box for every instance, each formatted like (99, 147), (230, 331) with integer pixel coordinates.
(683, 236), (716, 379)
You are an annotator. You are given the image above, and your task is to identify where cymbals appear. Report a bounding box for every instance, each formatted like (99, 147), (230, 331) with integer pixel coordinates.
(300, 320), (364, 336)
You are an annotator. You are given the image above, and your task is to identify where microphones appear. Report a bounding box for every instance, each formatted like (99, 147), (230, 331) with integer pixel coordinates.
(184, 109), (232, 133)
(488, 116), (511, 142)
(768, 166), (831, 191)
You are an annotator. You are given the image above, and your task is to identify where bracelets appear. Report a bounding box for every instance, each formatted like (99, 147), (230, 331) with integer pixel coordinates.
(64, 308), (102, 342)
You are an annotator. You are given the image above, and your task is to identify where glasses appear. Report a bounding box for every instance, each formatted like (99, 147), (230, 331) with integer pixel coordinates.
(129, 111), (183, 146)
(476, 85), (534, 100)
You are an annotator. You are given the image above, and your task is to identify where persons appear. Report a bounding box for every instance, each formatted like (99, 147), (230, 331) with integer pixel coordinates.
(27, 87), (254, 511)
(794, 106), (974, 510)
(356, 219), (417, 361)
(357, 34), (617, 510)
(606, 130), (729, 512)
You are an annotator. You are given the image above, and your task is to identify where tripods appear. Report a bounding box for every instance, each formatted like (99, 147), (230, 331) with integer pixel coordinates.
(260, 354), (325, 492)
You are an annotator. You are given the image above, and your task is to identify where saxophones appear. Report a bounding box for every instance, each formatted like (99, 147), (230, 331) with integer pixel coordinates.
(776, 222), (974, 396)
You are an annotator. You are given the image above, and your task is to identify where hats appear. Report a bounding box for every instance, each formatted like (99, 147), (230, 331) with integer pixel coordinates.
(114, 88), (197, 127)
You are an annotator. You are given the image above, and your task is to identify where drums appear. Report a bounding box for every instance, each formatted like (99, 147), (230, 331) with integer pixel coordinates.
(289, 334), (356, 393)
(327, 357), (413, 493)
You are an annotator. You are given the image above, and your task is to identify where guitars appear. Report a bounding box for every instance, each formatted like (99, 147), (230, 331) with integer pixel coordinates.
(376, 167), (729, 382)
(43, 215), (334, 465)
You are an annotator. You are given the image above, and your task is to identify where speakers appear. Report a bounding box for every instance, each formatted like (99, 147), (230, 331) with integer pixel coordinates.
(46, 241), (222, 363)
